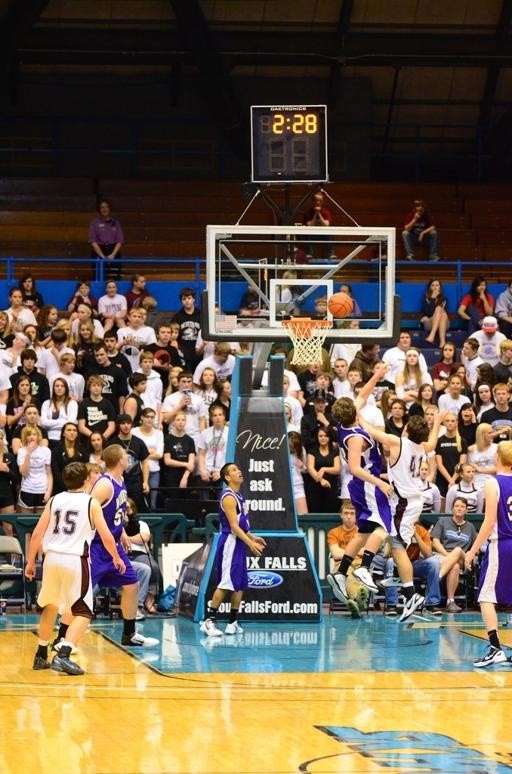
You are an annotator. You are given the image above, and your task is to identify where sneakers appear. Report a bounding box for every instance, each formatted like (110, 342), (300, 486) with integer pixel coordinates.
(473, 646), (506, 667)
(121, 632), (159, 646)
(33, 638), (84, 675)
(225, 620), (243, 634)
(135, 608), (144, 620)
(326, 567), (462, 622)
(200, 619), (223, 636)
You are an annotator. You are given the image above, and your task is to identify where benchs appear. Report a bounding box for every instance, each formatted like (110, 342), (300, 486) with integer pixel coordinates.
(0, 177), (512, 281)
(400, 329), (475, 372)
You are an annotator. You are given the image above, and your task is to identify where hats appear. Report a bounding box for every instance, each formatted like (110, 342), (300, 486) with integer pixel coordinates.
(482, 316), (497, 332)
(315, 389), (325, 402)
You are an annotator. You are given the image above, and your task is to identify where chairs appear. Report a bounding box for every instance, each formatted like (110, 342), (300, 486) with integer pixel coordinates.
(325, 574), (479, 616)
(0, 534), (28, 614)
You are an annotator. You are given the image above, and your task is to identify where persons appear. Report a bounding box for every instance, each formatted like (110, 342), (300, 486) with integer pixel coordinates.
(302, 187), (340, 261)
(198, 462), (267, 637)
(86, 195), (124, 282)
(272, 204), (300, 254)
(367, 237), (401, 285)
(87, 442), (146, 646)
(400, 196), (442, 264)
(1, 271), (512, 622)
(22, 460), (128, 677)
(464, 441), (512, 670)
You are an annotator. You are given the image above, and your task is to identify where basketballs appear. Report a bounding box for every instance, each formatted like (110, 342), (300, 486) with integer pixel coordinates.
(328, 293), (354, 317)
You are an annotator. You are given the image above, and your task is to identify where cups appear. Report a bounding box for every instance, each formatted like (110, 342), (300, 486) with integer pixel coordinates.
(181, 388), (192, 408)
